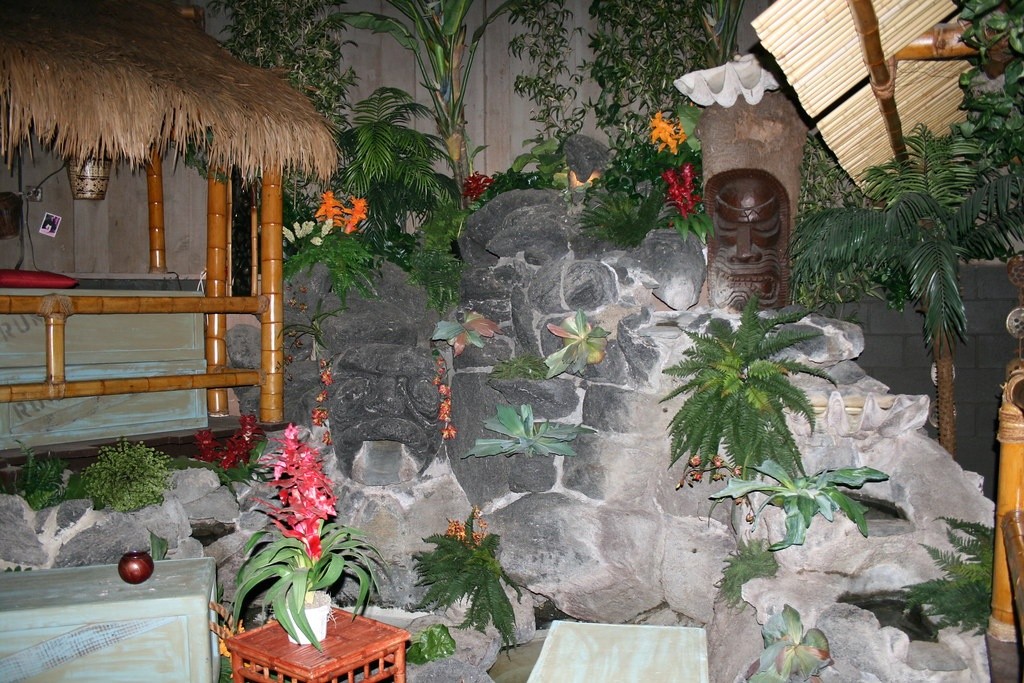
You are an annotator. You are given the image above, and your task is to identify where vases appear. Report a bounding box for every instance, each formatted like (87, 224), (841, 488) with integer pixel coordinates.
(283, 592), (333, 645)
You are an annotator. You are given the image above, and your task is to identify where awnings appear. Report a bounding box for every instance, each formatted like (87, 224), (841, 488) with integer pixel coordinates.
(750, 0), (977, 200)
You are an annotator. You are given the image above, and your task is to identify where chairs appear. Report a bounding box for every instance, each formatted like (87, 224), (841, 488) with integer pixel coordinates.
(0, 191), (79, 287)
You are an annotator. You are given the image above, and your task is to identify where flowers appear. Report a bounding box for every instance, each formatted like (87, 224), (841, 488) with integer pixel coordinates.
(218, 418), (388, 652)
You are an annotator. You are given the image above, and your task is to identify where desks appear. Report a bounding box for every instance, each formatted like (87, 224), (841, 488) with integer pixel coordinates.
(225, 607), (411, 683)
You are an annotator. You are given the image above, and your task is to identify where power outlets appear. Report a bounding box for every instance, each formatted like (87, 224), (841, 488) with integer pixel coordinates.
(24, 186), (43, 202)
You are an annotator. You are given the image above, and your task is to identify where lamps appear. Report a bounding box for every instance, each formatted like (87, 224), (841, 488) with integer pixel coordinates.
(67, 155), (109, 200)
